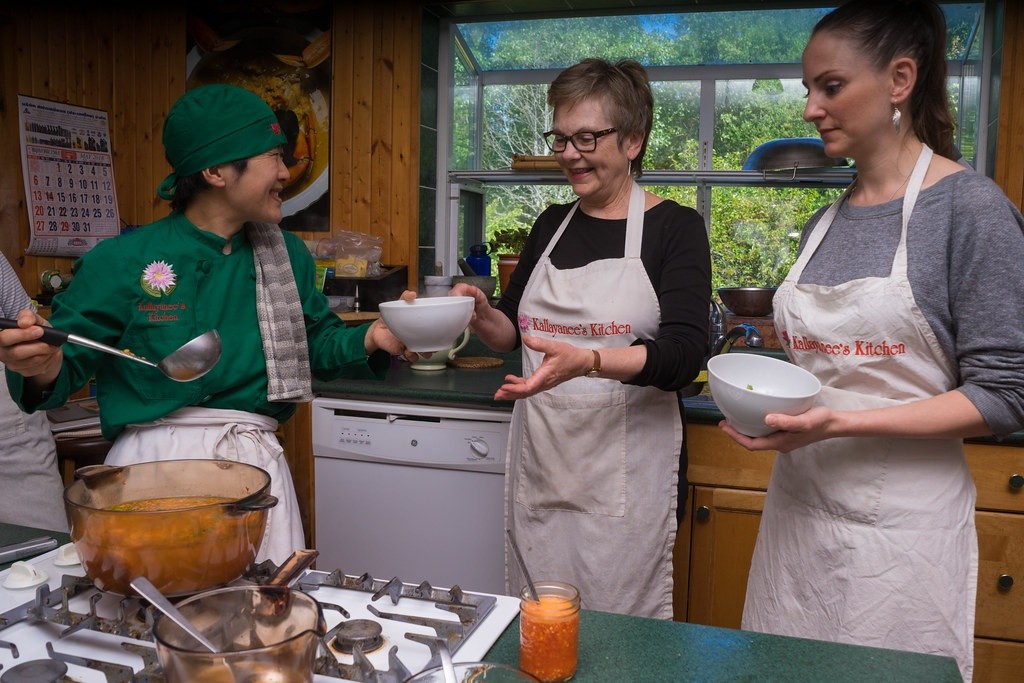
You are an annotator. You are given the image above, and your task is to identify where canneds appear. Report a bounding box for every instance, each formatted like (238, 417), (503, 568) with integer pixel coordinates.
(519, 580), (581, 681)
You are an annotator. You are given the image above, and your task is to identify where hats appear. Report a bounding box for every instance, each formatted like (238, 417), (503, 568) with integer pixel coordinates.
(163, 84), (287, 176)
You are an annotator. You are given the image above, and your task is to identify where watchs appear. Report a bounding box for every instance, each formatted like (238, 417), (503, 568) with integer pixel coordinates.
(586, 350), (601, 377)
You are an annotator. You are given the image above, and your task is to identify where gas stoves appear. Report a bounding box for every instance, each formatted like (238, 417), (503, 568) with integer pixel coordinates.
(1, 535), (523, 683)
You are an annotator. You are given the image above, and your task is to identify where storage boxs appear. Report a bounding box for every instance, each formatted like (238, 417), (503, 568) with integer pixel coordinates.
(322, 264), (410, 312)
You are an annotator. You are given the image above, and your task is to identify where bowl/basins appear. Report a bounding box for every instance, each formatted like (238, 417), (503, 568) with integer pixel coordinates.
(378, 296), (476, 353)
(404, 661), (544, 683)
(706, 352), (822, 437)
(717, 286), (778, 317)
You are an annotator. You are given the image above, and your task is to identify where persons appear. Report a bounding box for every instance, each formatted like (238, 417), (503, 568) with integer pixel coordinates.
(0, 84), (438, 566)
(446, 57), (712, 621)
(0, 251), (73, 571)
(717, 0), (1024, 683)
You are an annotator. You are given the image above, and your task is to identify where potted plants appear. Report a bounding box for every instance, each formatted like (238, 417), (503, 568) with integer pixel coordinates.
(488, 228), (530, 294)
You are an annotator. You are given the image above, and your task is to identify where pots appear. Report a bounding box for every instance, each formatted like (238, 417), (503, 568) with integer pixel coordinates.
(61, 455), (279, 599)
(153, 548), (331, 683)
(743, 137), (851, 183)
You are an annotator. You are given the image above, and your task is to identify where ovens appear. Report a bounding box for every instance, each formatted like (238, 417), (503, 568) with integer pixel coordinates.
(308, 397), (514, 601)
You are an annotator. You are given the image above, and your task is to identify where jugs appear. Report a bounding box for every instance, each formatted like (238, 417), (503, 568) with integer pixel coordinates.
(399, 325), (470, 370)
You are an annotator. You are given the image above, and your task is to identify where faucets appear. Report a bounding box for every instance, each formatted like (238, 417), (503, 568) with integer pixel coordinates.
(709, 299), (764, 358)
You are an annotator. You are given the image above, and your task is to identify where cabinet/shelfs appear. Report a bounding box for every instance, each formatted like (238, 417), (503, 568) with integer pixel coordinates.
(679, 420), (1024, 683)
(274, 400), (315, 550)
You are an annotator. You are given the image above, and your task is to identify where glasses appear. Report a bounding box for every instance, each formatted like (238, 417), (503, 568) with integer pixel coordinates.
(543, 126), (620, 153)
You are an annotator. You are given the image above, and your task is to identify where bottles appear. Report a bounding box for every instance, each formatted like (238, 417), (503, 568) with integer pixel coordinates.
(466, 245), (492, 277)
(518, 579), (582, 683)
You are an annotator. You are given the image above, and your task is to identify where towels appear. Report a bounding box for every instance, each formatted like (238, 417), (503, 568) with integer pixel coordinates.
(243, 222), (315, 404)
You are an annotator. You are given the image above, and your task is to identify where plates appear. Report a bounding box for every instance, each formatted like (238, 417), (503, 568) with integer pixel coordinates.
(184, 45), (331, 218)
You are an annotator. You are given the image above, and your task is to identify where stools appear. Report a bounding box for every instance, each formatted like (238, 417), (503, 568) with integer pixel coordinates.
(58, 439), (113, 486)
